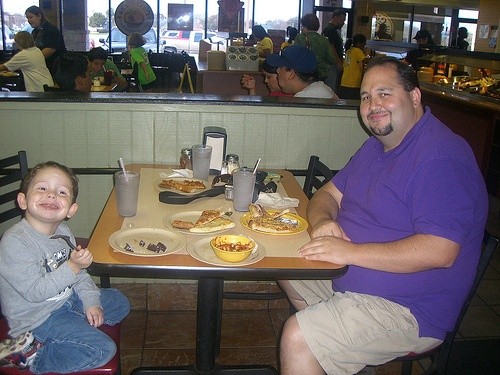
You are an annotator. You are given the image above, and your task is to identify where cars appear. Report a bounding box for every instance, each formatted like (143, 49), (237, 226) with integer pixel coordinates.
(98, 24), (166, 53)
(155, 26), (230, 54)
(0, 22), (34, 51)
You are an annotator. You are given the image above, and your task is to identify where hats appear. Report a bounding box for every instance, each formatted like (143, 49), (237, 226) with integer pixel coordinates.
(412, 30), (430, 39)
(266, 45), (316, 73)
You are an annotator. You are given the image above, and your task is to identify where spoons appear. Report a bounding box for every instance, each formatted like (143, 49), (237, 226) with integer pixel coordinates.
(53, 235), (79, 252)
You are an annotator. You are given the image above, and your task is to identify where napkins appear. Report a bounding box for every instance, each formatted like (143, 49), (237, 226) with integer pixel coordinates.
(255, 191), (299, 207)
(168, 168), (194, 179)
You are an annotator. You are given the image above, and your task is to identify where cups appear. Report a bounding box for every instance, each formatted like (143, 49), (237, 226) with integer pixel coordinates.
(115, 171), (140, 217)
(93, 79), (101, 86)
(232, 168), (256, 212)
(103, 70), (113, 86)
(192, 144), (213, 181)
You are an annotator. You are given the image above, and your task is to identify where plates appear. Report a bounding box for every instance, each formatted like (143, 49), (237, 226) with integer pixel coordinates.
(240, 210), (309, 235)
(163, 210), (235, 235)
(108, 227), (187, 257)
(153, 177), (211, 196)
(186, 236), (266, 267)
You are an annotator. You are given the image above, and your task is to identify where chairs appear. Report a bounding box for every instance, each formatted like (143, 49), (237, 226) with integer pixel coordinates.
(0, 48), (174, 93)
(0, 151), (118, 375)
(305, 156), (500, 375)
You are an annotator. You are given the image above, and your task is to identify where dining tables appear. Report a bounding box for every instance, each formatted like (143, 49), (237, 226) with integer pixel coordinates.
(85, 163), (348, 375)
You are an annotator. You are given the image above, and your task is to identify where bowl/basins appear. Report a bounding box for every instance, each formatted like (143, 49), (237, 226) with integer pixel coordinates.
(209, 233), (256, 263)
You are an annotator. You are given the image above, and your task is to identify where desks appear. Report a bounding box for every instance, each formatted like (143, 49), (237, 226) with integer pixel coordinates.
(194, 54), (208, 93)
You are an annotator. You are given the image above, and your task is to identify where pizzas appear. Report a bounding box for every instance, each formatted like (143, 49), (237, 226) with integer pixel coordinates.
(171, 209), (236, 232)
(249, 203), (300, 233)
(160, 179), (206, 193)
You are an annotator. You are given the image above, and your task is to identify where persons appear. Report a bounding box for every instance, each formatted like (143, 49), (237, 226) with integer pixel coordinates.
(82, 47), (127, 89)
(276, 56), (489, 375)
(266, 45), (340, 99)
(293, 13), (338, 83)
(127, 32), (157, 93)
(280, 26), (297, 51)
(401, 30), (437, 72)
(245, 25), (274, 57)
(241, 6), (369, 99)
(53, 51), (93, 93)
(450, 27), (469, 52)
(25, 6), (67, 66)
(321, 9), (347, 91)
(339, 35), (370, 99)
(0, 31), (55, 93)
(374, 24), (393, 41)
(241, 59), (294, 97)
(0, 161), (131, 375)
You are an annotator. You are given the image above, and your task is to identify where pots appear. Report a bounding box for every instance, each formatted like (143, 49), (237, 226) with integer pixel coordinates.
(416, 66), (434, 84)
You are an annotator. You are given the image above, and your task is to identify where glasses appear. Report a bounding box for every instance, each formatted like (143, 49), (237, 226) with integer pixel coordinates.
(300, 25), (305, 29)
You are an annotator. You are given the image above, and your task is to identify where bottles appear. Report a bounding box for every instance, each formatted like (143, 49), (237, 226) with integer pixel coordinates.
(221, 153), (240, 175)
(179, 148), (192, 171)
